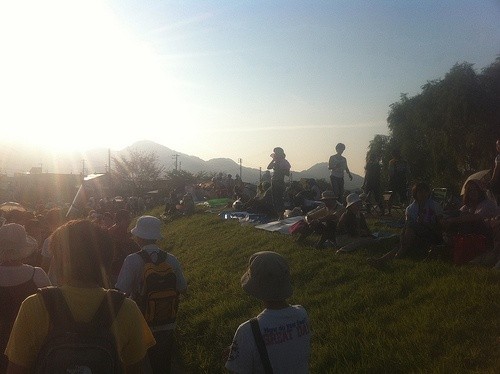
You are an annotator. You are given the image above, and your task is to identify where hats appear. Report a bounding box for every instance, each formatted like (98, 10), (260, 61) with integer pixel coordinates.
(346, 193), (362, 208)
(0, 223), (37, 260)
(321, 190), (340, 199)
(130, 216), (162, 239)
(241, 250), (293, 299)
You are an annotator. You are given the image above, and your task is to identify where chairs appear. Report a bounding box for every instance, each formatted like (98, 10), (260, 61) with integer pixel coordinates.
(381, 191), (393, 217)
(432, 188), (447, 205)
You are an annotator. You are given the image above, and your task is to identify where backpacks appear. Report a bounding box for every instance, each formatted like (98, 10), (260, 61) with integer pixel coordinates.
(34, 286), (127, 374)
(137, 249), (178, 326)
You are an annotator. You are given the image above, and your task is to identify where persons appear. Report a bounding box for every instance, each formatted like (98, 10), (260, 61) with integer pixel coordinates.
(360, 153), (389, 216)
(1, 223), (53, 373)
(267, 148), (291, 209)
(389, 149), (409, 207)
(225, 251), (312, 374)
(109, 210), (142, 291)
(4, 219), (158, 373)
(328, 144), (353, 196)
(114, 215), (188, 374)
(0, 169), (500, 286)
(488, 139), (500, 206)
